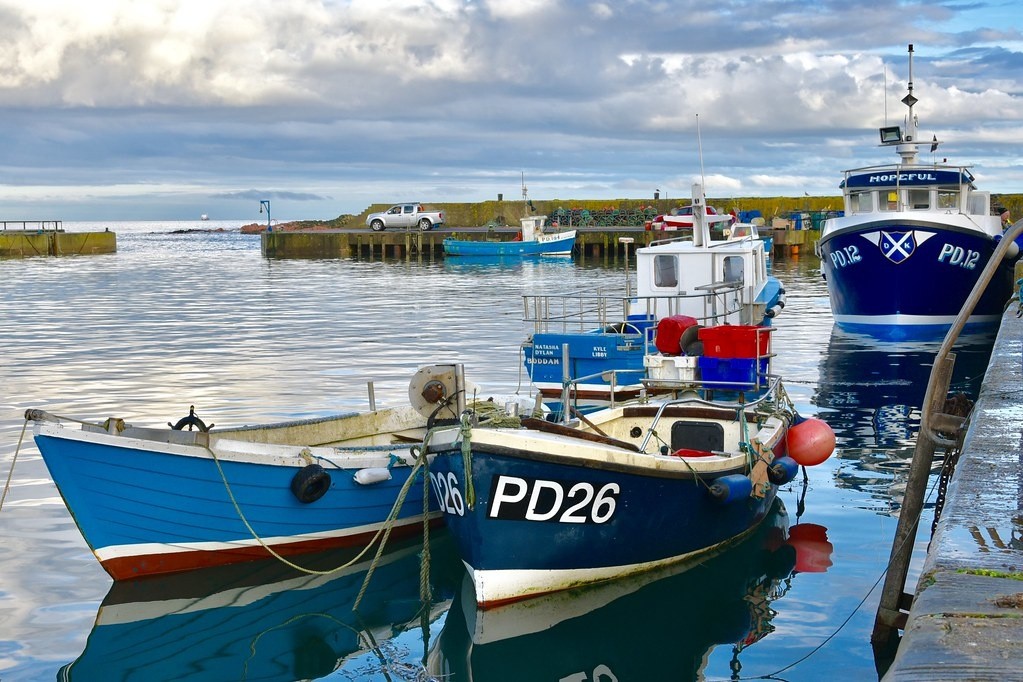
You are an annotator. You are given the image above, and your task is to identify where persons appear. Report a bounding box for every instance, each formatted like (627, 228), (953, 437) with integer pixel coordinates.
(997, 207), (1014, 230)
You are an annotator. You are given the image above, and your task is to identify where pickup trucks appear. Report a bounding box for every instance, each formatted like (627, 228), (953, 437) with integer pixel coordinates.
(364, 200), (446, 232)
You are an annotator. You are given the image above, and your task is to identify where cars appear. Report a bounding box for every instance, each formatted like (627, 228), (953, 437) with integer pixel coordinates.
(653, 204), (718, 230)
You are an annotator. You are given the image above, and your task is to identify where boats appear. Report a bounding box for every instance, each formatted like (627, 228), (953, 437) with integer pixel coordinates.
(412, 326), (804, 611)
(515, 110), (790, 421)
(48, 523), (464, 682)
(430, 494), (793, 681)
(442, 170), (577, 256)
(814, 42), (1023, 326)
(22, 375), (550, 581)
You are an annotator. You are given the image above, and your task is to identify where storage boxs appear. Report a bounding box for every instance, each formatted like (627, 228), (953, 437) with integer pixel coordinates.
(626, 314), (658, 341)
(671, 449), (716, 458)
(695, 324), (769, 390)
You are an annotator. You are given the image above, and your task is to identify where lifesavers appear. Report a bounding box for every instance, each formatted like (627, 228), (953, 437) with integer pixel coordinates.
(288, 463), (332, 505)
